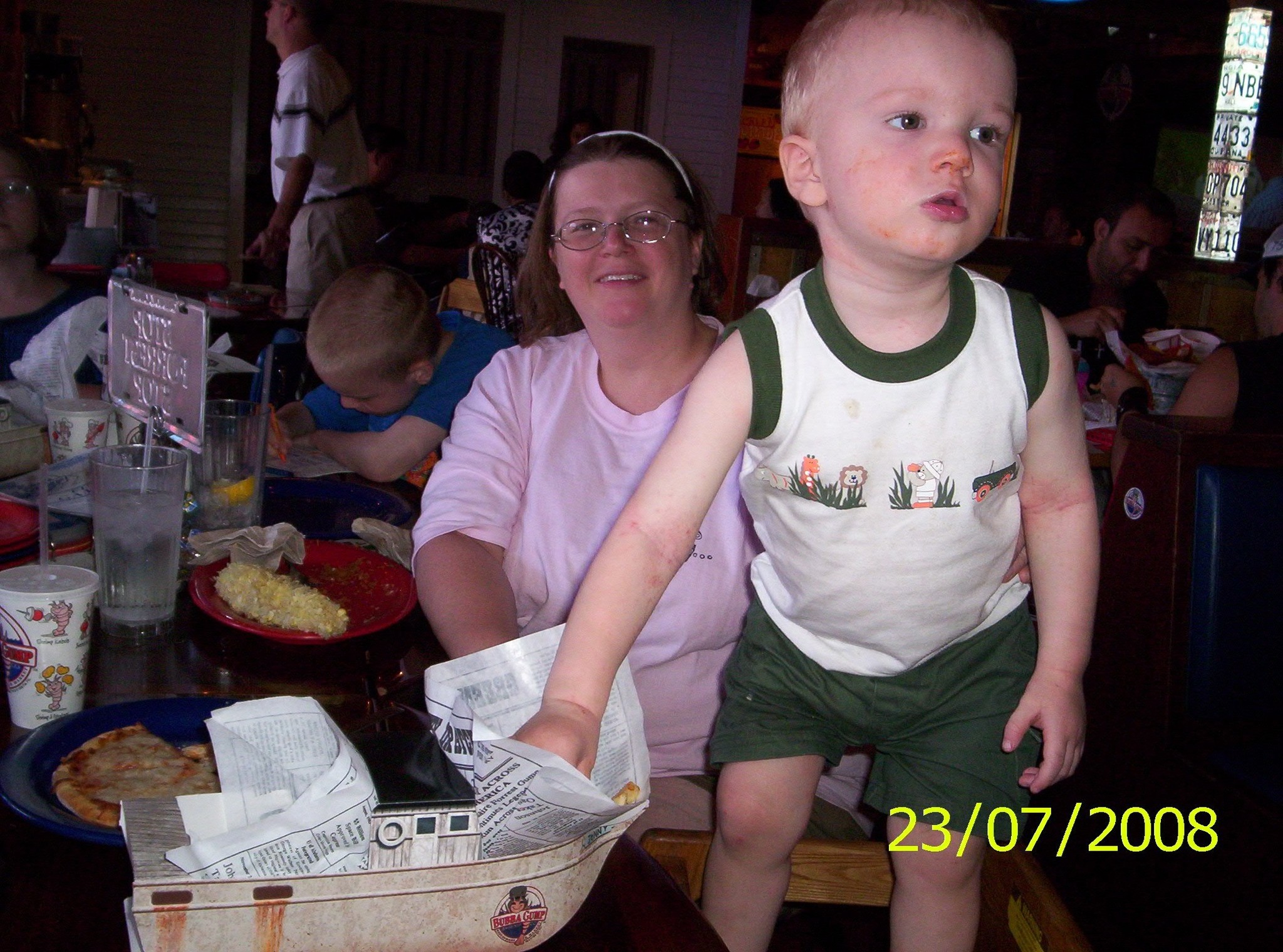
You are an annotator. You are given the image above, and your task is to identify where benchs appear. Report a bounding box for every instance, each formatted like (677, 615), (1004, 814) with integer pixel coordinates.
(717, 214), (1283, 952)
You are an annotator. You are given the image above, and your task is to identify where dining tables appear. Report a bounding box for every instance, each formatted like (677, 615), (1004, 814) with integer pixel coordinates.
(0, 473), (732, 952)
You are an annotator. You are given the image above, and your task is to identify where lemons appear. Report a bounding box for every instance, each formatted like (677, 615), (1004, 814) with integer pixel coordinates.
(211, 475), (257, 507)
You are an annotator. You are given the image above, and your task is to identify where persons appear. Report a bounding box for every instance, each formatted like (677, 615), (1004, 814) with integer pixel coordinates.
(1002, 180), (1179, 392)
(1097, 224), (1282, 491)
(410, 129), (877, 848)
(266, 260), (517, 484)
(246, 0), (378, 319)
(478, 112), (600, 340)
(507, 0), (1101, 951)
(0, 133), (108, 400)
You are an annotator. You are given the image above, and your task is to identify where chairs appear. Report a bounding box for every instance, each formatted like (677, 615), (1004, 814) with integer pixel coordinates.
(472, 244), (521, 344)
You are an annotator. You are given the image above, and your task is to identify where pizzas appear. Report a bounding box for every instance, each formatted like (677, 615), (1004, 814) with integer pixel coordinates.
(51, 722), (221, 826)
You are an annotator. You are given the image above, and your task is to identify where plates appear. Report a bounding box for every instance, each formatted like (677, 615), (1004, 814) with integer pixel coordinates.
(0, 697), (245, 846)
(260, 477), (411, 541)
(188, 539), (418, 645)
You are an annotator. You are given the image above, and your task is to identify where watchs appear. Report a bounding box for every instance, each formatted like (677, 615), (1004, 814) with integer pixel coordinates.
(1115, 387), (1149, 422)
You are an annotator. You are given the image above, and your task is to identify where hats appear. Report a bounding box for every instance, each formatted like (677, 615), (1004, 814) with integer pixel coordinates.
(1262, 223), (1283, 258)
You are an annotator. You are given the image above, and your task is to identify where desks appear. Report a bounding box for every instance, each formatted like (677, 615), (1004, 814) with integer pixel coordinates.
(180, 290), (309, 401)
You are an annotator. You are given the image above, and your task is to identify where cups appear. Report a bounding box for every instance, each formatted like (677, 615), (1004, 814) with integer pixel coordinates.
(1179, 329), (1220, 357)
(89, 444), (187, 639)
(112, 402), (166, 445)
(190, 399), (271, 532)
(43, 398), (114, 463)
(0, 564), (99, 730)
(1142, 329), (1182, 349)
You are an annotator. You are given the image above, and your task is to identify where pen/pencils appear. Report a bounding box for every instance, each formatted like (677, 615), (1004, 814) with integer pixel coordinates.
(267, 404), (287, 463)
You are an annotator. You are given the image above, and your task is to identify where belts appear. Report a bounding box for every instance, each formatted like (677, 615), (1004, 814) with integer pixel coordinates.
(309, 187), (363, 204)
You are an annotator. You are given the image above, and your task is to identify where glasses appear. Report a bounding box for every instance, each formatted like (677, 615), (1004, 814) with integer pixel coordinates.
(0, 181), (35, 203)
(549, 210), (693, 253)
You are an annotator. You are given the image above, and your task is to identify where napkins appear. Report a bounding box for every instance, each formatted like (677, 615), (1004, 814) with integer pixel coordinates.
(187, 521), (307, 574)
(352, 517), (415, 572)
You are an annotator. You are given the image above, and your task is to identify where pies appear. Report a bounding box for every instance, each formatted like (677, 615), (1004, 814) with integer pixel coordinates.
(612, 781), (641, 805)
